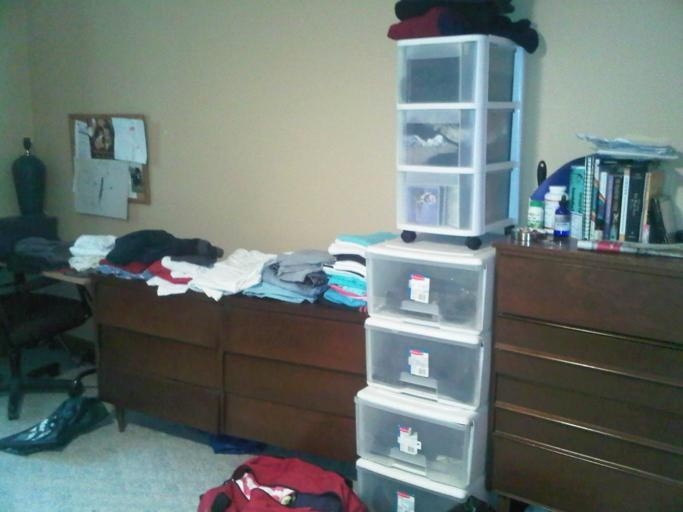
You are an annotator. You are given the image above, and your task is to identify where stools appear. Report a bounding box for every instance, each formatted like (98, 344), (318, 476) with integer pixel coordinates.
(0, 275), (95, 420)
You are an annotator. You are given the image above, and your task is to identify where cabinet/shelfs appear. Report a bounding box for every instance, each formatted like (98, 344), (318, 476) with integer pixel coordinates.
(484, 231), (683, 509)
(224, 293), (364, 464)
(394, 32), (525, 248)
(94, 280), (222, 452)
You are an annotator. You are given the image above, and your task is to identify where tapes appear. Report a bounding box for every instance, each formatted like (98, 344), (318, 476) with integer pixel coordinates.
(510, 227), (538, 241)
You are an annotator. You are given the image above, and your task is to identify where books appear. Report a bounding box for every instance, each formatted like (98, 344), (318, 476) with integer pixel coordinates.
(570, 152), (666, 243)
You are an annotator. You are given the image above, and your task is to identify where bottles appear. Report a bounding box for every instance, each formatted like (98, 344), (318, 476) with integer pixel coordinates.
(526, 185), (571, 237)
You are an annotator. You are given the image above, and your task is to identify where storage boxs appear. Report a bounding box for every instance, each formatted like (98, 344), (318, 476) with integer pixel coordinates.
(355, 239), (497, 512)
